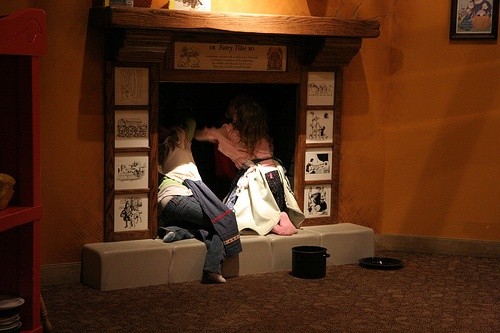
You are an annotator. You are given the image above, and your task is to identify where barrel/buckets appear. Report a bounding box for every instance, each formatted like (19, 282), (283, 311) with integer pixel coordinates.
(292, 245), (330, 278)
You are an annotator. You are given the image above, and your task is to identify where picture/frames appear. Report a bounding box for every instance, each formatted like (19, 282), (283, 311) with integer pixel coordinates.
(449, 0), (499, 40)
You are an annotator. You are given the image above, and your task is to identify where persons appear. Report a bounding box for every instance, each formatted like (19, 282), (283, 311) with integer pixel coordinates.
(193, 96), (305, 235)
(156, 119), (242, 283)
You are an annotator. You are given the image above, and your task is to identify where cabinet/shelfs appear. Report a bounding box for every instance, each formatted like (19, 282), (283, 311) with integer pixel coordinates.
(0, 6), (47, 333)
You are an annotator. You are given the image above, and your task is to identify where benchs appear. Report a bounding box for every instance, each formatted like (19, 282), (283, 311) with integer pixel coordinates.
(80, 222), (376, 292)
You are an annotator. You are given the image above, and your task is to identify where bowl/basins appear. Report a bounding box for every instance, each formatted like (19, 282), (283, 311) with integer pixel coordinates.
(0, 173), (16, 210)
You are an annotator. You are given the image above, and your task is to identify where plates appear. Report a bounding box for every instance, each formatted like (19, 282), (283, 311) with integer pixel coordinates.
(359, 256), (404, 269)
(0, 296), (25, 333)
